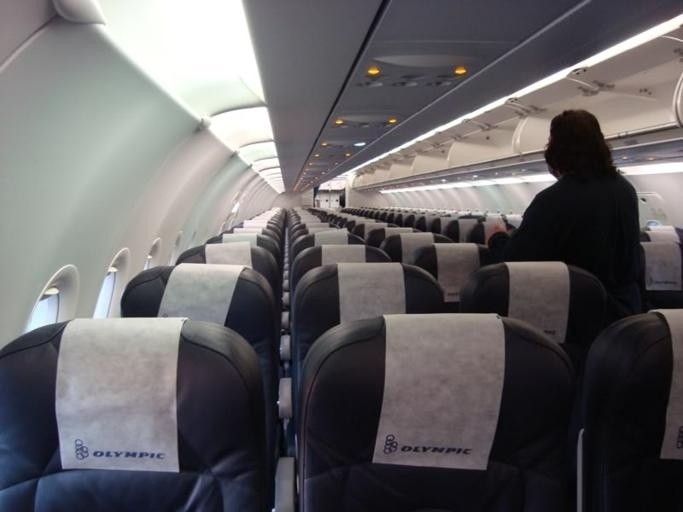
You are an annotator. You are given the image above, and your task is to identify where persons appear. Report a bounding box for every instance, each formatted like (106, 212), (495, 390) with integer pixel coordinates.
(484, 108), (648, 328)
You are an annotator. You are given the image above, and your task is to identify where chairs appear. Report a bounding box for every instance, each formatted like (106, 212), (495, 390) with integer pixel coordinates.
(273, 312), (576, 511)
(0, 315), (271, 510)
(577, 307), (682, 510)
(117, 204), (683, 455)
